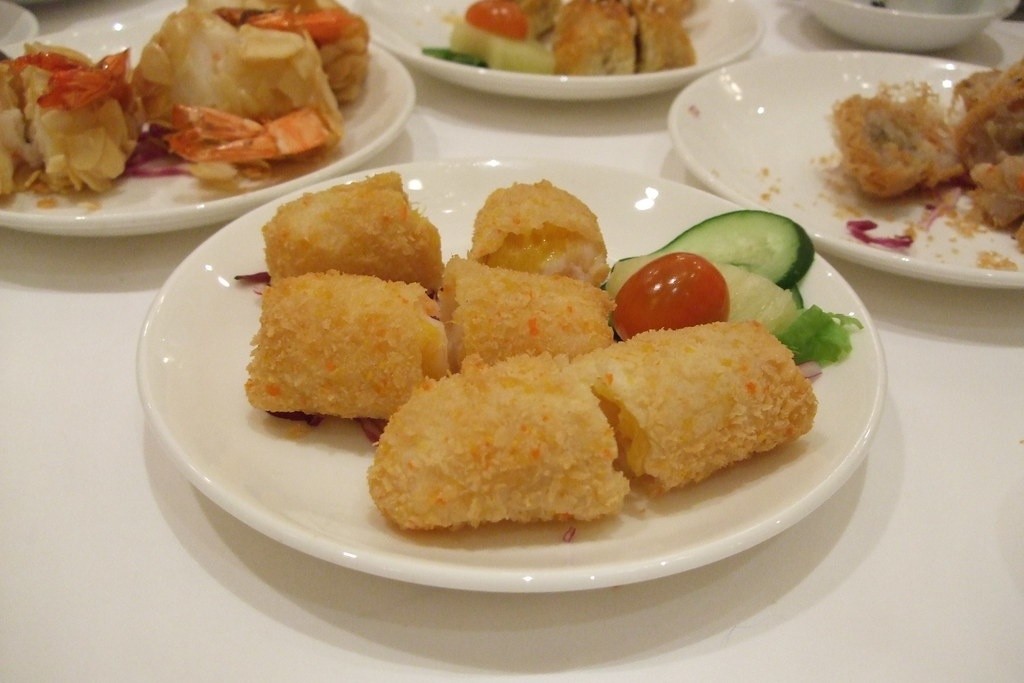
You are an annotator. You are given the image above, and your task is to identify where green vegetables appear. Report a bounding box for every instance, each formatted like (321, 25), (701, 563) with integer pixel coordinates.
(423, 48), (491, 67)
(771, 304), (865, 367)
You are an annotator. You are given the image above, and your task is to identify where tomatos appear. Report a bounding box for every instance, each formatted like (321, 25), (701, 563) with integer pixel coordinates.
(466, 0), (529, 42)
(611, 251), (728, 341)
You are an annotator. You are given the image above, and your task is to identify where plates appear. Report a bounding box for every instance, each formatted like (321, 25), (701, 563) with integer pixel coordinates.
(338, 1), (767, 100)
(666, 52), (1024, 290)
(0, 1), (41, 47)
(0, 10), (418, 238)
(137, 157), (886, 593)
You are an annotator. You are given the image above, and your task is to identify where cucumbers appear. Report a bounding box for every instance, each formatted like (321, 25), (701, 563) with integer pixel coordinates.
(653, 210), (816, 289)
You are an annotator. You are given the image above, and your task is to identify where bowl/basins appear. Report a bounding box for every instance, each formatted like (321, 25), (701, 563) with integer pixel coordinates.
(807, 1), (1018, 51)
